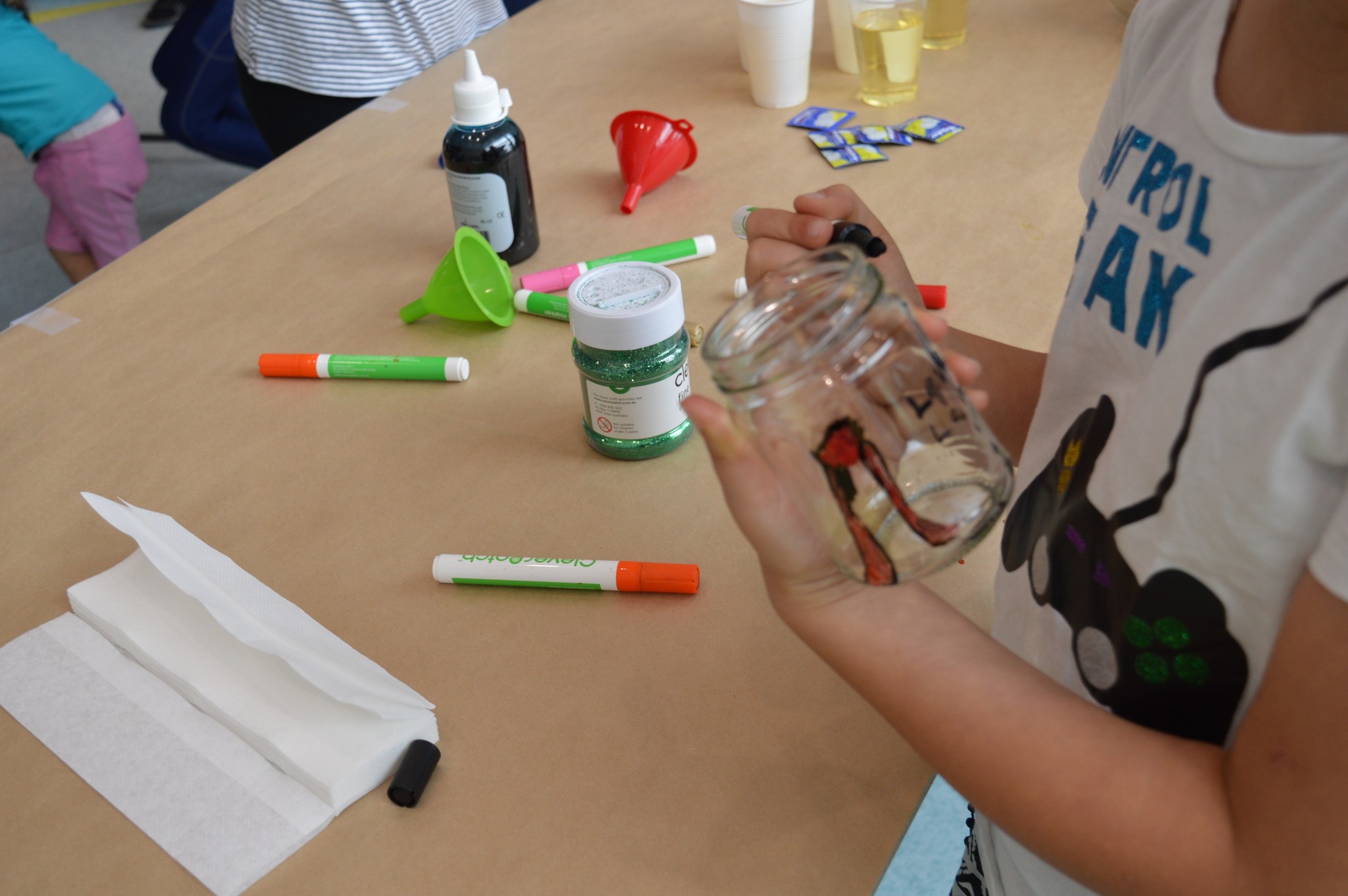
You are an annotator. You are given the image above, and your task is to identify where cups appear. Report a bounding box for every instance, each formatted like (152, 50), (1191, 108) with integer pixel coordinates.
(826, 0), (896, 75)
(847, 0), (926, 109)
(739, 0), (815, 109)
(896, 0), (968, 51)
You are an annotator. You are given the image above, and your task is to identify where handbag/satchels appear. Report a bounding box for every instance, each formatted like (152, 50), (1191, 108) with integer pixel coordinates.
(152, 0), (276, 168)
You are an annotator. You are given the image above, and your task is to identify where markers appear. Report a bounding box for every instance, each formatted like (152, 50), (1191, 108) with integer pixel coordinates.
(431, 554), (700, 593)
(520, 235), (715, 295)
(257, 353), (470, 382)
(735, 276), (946, 310)
(732, 206), (889, 260)
(513, 289), (705, 347)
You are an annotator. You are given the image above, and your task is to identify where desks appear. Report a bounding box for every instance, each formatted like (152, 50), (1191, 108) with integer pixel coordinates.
(0, 0), (1133, 896)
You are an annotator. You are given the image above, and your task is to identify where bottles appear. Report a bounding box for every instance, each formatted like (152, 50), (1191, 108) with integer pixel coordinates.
(441, 49), (540, 267)
(698, 240), (1016, 588)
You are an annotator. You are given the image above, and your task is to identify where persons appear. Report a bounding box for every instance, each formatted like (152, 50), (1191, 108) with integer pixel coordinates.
(672, 2), (1348, 895)
(228, 1), (511, 166)
(0, 0), (150, 285)
